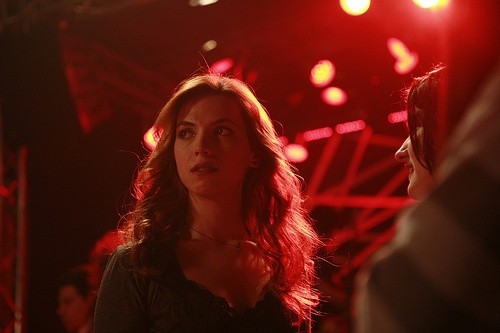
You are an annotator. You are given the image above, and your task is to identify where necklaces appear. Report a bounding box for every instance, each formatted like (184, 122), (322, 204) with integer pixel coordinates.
(185, 222), (247, 251)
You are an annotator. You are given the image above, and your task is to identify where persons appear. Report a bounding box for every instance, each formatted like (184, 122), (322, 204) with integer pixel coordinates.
(55, 230), (132, 333)
(94, 51), (325, 333)
(351, 62), (500, 333)
